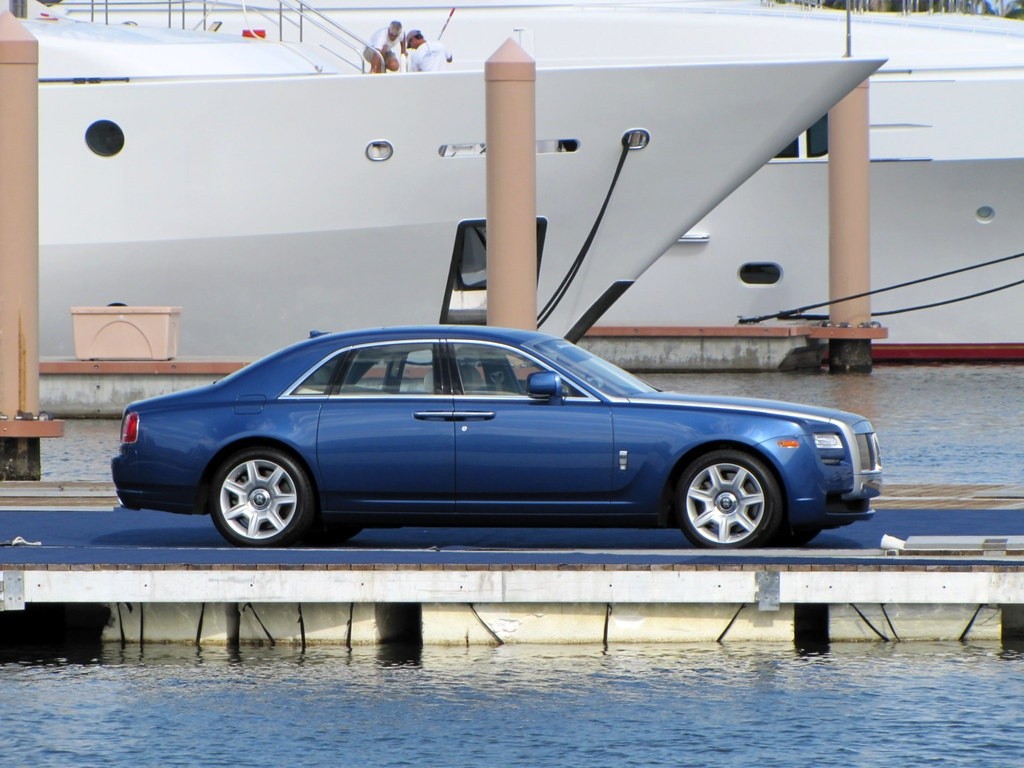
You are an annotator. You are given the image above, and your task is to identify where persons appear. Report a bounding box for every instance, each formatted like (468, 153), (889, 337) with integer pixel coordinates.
(363, 21), (408, 73)
(406, 29), (452, 71)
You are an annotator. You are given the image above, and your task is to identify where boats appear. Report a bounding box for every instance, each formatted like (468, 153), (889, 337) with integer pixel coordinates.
(593, 0), (1024, 361)
(2, 0), (888, 366)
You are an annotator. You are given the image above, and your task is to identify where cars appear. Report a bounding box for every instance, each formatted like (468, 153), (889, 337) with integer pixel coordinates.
(110, 324), (882, 550)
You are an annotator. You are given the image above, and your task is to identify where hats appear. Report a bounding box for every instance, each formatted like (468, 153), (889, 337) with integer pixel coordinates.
(407, 29), (420, 48)
